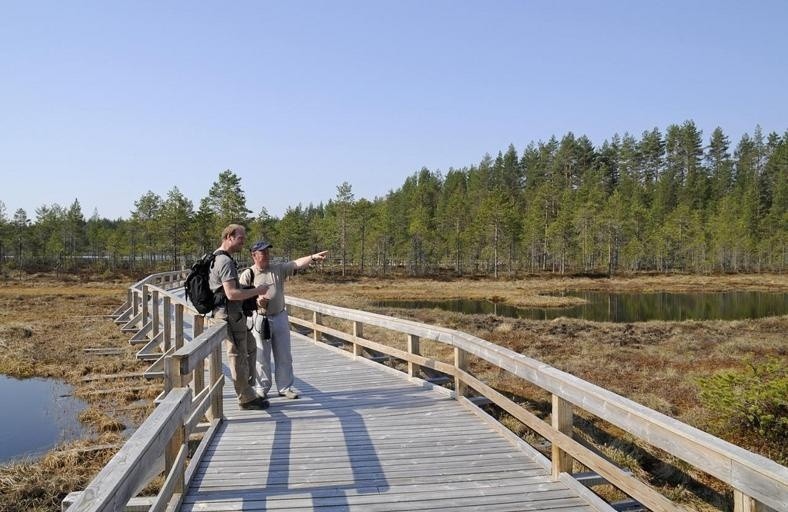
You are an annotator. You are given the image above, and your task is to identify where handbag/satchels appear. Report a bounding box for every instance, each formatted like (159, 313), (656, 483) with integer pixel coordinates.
(242, 284), (259, 317)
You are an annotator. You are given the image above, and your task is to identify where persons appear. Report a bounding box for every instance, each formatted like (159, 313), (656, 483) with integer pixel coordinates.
(207, 224), (270, 411)
(239, 240), (330, 401)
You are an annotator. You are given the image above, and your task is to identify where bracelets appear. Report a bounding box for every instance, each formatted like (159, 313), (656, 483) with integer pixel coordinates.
(311, 255), (317, 261)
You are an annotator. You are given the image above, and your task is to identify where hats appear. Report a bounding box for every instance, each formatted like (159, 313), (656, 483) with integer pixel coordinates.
(249, 241), (274, 252)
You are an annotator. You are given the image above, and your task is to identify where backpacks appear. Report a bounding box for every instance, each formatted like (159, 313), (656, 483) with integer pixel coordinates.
(183, 249), (238, 316)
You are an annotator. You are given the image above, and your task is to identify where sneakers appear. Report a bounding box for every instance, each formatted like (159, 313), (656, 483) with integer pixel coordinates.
(237, 396), (271, 411)
(277, 385), (301, 399)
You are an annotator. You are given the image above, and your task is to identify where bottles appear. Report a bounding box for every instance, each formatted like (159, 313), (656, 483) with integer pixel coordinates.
(260, 316), (270, 341)
